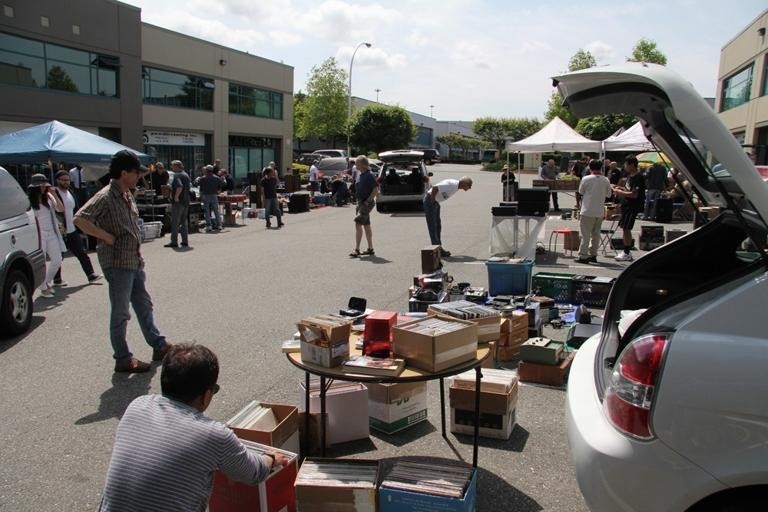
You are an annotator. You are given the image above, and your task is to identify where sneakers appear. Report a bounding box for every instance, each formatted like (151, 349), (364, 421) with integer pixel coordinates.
(164, 240), (189, 248)
(153, 344), (172, 360)
(440, 248), (451, 258)
(88, 274), (104, 283)
(204, 224), (223, 232)
(575, 254), (598, 264)
(41, 278), (68, 298)
(114, 356), (151, 374)
(615, 250), (634, 262)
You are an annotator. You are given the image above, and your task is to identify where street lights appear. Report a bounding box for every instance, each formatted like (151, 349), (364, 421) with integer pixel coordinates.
(347, 40), (372, 157)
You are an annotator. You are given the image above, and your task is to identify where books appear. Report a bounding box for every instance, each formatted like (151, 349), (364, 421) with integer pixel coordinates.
(300, 377), (369, 448)
(229, 400), (299, 455)
(428, 303), (501, 343)
(364, 382), (427, 434)
(449, 368), (515, 440)
(379, 461), (478, 512)
(294, 313), (351, 369)
(392, 314), (478, 373)
(296, 459), (378, 512)
(282, 341), (300, 353)
(342, 354), (405, 376)
(209, 438), (298, 512)
(294, 331), (300, 339)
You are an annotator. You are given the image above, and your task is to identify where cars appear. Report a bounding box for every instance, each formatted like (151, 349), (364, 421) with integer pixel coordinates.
(291, 148), (414, 181)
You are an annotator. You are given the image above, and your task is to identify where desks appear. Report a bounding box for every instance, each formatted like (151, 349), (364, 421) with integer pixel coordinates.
(283, 321), (495, 474)
(133, 198), (201, 241)
(213, 193), (248, 228)
(491, 214), (547, 262)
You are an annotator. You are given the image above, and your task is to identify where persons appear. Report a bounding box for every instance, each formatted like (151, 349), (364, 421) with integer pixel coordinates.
(97, 344), (287, 511)
(50, 169), (104, 286)
(26, 174), (62, 298)
(350, 155), (379, 256)
(423, 177), (473, 257)
(502, 157), (686, 264)
(69, 158), (361, 248)
(73, 151), (172, 373)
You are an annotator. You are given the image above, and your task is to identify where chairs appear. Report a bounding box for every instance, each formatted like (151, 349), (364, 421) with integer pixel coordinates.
(597, 213), (625, 257)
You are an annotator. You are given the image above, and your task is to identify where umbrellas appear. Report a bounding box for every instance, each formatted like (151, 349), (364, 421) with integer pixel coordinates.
(636, 153), (672, 167)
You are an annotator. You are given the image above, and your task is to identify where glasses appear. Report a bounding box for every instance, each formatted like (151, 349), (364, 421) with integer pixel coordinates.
(207, 383), (220, 394)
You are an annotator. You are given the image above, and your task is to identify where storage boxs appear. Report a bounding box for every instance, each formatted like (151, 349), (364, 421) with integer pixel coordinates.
(377, 455), (480, 512)
(298, 376), (371, 450)
(293, 455), (381, 512)
(362, 377), (428, 435)
(297, 312), (351, 371)
(210, 439), (299, 511)
(392, 261), (575, 386)
(221, 400), (299, 473)
(449, 368), (519, 441)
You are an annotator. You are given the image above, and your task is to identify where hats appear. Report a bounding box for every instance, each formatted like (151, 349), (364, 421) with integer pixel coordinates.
(503, 165), (508, 170)
(28, 173), (51, 189)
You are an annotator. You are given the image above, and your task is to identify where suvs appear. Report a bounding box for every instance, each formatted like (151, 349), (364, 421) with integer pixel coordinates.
(549, 62), (768, 512)
(423, 149), (441, 164)
(375, 150), (434, 211)
(0, 167), (47, 339)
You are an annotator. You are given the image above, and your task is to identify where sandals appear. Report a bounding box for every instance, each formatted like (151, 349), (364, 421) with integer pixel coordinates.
(350, 247), (375, 257)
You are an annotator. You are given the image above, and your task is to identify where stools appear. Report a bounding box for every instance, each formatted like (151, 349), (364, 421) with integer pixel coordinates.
(548, 229), (573, 260)
